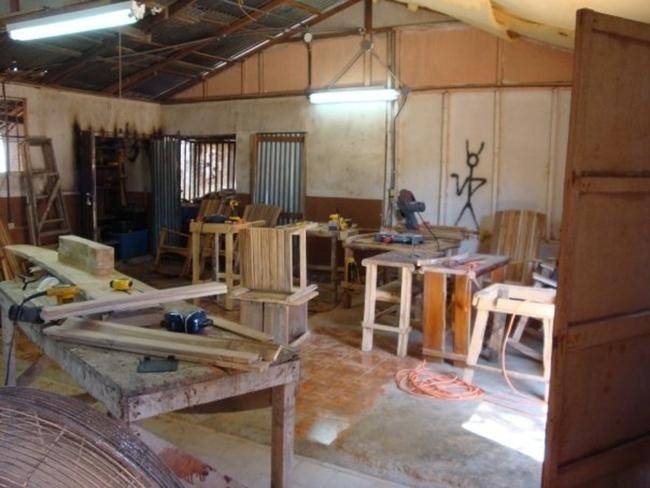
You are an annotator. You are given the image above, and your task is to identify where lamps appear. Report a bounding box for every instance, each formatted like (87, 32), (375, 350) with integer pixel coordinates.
(5, 0), (145, 41)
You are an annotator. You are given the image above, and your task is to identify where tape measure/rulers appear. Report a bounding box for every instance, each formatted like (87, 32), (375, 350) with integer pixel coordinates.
(109, 278), (133, 290)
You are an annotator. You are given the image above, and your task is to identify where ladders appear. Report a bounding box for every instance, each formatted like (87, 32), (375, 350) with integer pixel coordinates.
(19, 136), (74, 246)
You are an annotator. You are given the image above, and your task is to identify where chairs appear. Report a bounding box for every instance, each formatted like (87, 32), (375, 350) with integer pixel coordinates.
(153, 196), (283, 281)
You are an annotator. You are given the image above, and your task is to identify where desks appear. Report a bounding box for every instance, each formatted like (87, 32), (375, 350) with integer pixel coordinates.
(1, 268), (302, 487)
(275, 221), (508, 369)
